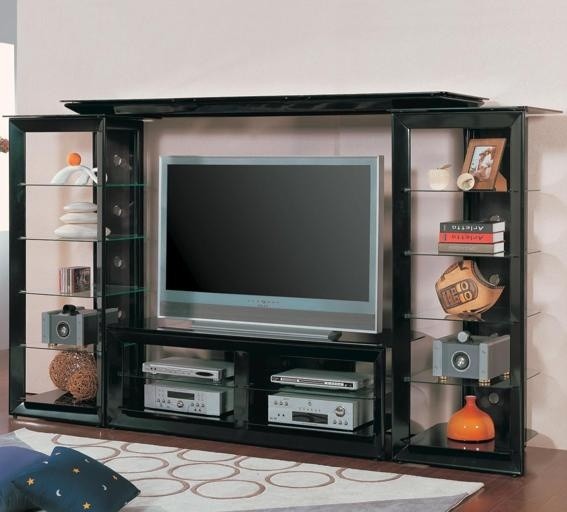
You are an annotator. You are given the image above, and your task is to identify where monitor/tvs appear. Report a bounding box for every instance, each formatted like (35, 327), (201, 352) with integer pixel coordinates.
(156, 154), (387, 341)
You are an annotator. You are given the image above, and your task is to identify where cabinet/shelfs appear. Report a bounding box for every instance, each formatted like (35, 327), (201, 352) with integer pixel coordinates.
(11, 113), (116, 423)
(112, 313), (383, 462)
(396, 113), (521, 471)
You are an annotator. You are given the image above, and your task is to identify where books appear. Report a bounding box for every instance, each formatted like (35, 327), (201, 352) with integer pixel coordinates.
(438, 230), (505, 245)
(437, 242), (505, 255)
(439, 220), (506, 234)
(57, 265), (91, 294)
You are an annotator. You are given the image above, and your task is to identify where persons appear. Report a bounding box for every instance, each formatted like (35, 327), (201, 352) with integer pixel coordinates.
(470, 151), (486, 179)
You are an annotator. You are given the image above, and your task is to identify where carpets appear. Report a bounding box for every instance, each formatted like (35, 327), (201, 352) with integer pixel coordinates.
(0, 426), (485, 512)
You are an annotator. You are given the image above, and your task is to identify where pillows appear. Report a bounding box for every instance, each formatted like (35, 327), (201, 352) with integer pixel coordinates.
(0, 445), (140, 512)
